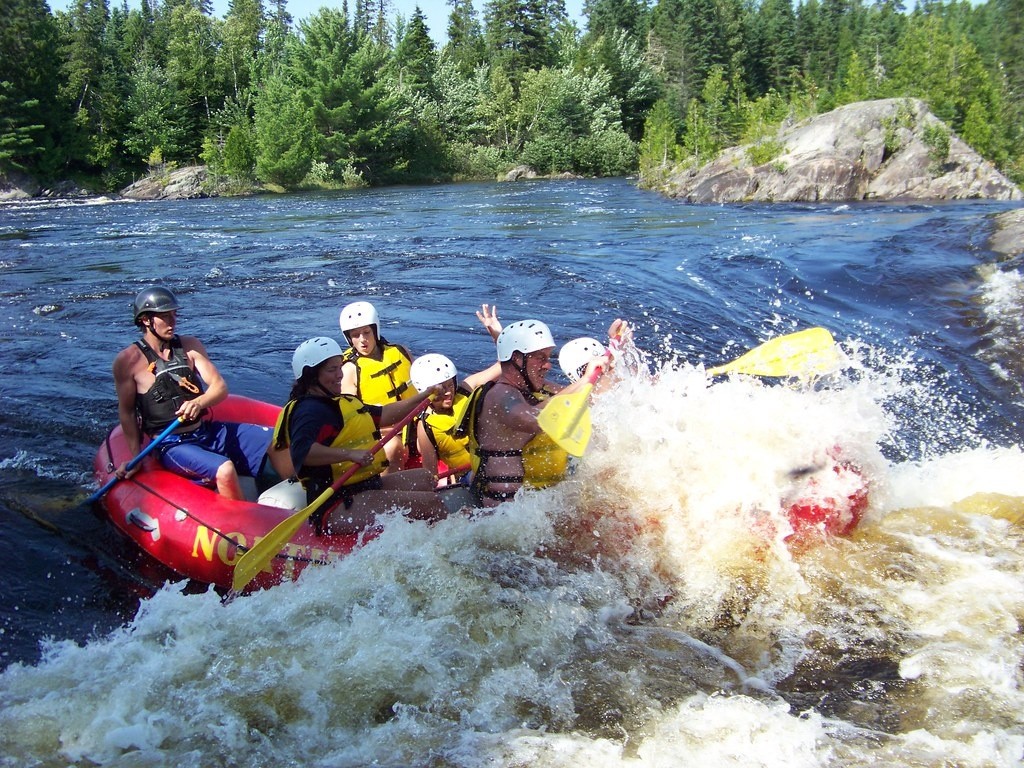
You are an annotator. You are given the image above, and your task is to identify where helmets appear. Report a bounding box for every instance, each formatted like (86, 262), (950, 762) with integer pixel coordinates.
(340, 302), (381, 347)
(292, 337), (344, 381)
(558, 336), (606, 377)
(411, 353), (460, 394)
(497, 319), (558, 362)
(133, 287), (184, 325)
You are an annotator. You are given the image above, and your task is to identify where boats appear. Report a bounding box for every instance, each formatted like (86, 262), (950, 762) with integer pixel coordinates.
(93, 393), (869, 595)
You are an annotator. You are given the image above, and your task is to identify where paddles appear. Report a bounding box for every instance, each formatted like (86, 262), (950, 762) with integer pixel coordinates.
(708, 327), (846, 381)
(76, 413), (188, 510)
(234, 391), (444, 592)
(536, 331), (621, 458)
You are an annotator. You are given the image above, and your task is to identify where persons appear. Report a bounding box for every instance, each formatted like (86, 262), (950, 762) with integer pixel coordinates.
(337, 302), (420, 474)
(287, 336), (444, 537)
(408, 302), (503, 486)
(466, 319), (633, 512)
(110, 286), (294, 502)
